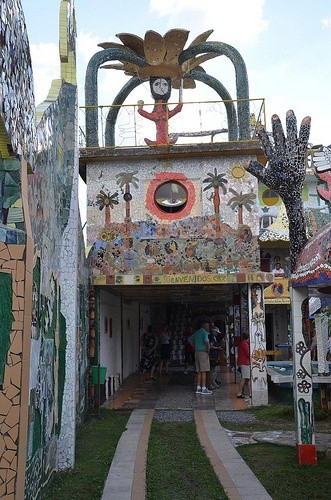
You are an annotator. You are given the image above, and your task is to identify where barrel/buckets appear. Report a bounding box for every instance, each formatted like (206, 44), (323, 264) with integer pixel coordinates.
(91, 365), (107, 385)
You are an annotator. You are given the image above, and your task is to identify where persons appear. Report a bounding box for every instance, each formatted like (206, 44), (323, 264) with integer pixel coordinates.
(236, 333), (252, 398)
(272, 263), (285, 276)
(140, 325), (172, 381)
(183, 322), (224, 394)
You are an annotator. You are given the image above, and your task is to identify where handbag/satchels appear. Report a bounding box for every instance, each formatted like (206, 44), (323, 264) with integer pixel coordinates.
(139, 357), (151, 372)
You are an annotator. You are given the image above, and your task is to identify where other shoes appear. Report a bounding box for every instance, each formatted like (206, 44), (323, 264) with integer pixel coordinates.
(150, 376), (155, 381)
(146, 376), (149, 381)
(209, 382), (220, 390)
(195, 387), (213, 394)
(237, 394), (244, 399)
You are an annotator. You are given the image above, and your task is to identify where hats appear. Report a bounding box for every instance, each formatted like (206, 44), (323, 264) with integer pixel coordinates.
(211, 327), (221, 334)
